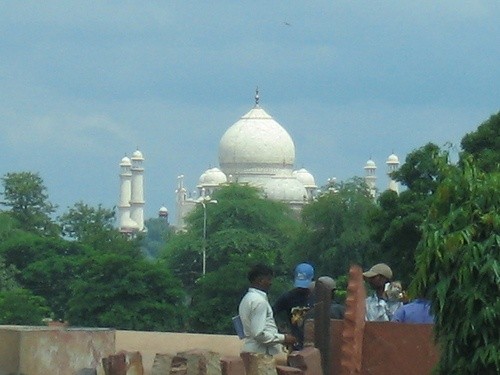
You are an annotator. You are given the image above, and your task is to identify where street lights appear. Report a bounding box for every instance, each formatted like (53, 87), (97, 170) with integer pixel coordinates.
(183, 188), (218, 279)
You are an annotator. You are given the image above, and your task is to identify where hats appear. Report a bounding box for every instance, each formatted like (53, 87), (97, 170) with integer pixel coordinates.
(362, 263), (393, 280)
(317, 277), (335, 291)
(293, 263), (314, 288)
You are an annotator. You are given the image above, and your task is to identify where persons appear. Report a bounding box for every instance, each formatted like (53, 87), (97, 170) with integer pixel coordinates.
(362, 263), (403, 322)
(238, 261), (296, 375)
(273, 263), (320, 347)
(393, 284), (437, 323)
(303, 276), (347, 331)
